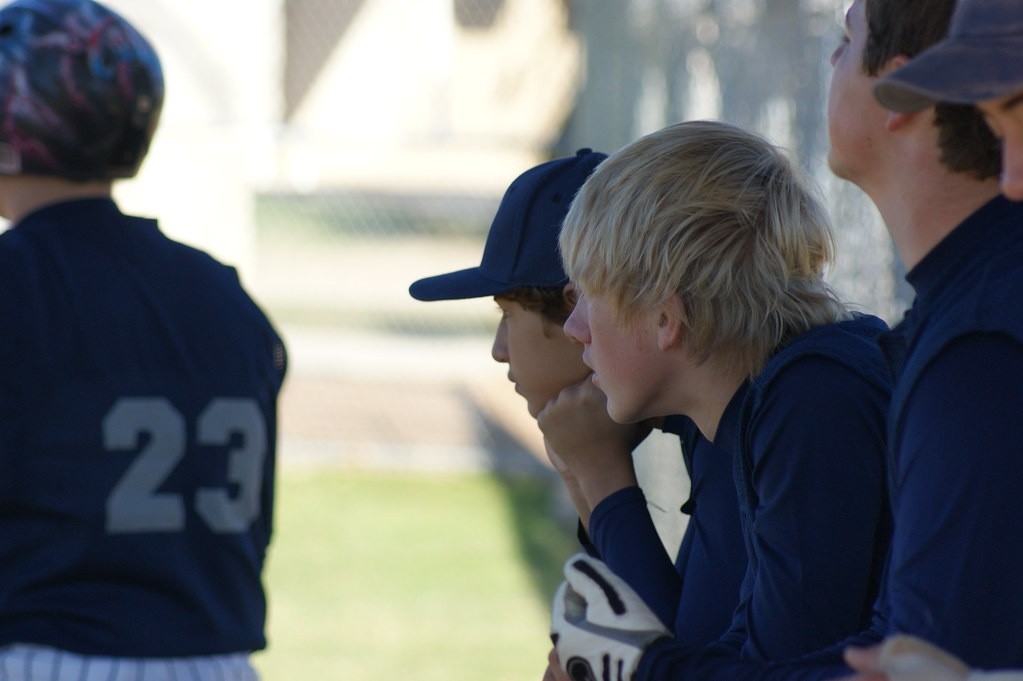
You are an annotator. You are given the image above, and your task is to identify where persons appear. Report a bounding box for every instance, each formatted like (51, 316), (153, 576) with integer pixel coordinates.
(407, 0), (1023, 681)
(0, 0), (288, 681)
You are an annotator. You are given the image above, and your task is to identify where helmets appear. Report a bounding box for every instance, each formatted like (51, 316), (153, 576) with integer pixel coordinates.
(0, 0), (165, 178)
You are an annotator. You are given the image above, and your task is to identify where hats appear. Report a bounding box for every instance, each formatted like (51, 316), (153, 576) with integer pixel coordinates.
(409, 147), (611, 303)
(873, 0), (1023, 113)
